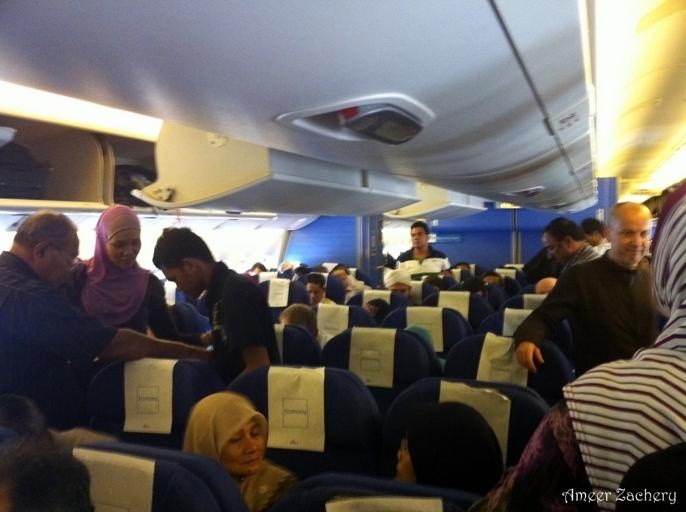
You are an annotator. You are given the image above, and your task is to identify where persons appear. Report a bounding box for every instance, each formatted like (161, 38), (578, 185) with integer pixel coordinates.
(0, 182), (686, 512)
